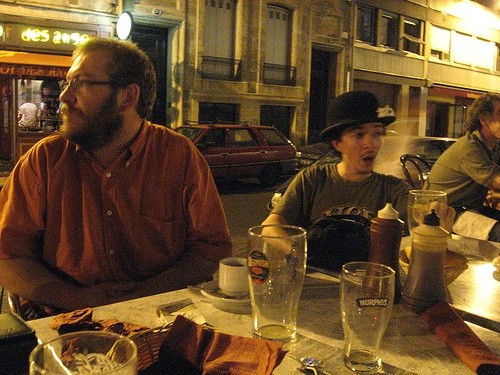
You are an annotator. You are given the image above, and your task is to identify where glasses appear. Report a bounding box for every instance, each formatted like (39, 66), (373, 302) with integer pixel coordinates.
(57, 79), (112, 92)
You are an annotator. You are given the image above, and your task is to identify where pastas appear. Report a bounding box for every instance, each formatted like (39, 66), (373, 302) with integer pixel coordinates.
(68, 353), (118, 375)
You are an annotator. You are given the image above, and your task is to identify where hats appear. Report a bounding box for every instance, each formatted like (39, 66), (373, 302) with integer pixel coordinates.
(319, 90), (396, 142)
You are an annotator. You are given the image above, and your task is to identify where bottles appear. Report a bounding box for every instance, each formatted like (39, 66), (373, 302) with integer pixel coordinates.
(369, 194), (404, 304)
(403, 209), (447, 316)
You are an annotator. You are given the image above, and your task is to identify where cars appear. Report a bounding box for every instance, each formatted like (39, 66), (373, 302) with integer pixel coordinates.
(170, 121), (297, 190)
(298, 130), (399, 170)
(267, 136), (459, 214)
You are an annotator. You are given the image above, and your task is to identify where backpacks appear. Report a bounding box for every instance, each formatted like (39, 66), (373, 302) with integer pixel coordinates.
(303, 214), (374, 276)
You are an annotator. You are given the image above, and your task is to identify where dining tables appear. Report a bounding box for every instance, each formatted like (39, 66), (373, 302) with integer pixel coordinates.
(25, 234), (500, 375)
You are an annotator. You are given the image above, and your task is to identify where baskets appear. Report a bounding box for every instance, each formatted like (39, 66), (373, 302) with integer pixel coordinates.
(125, 319), (225, 375)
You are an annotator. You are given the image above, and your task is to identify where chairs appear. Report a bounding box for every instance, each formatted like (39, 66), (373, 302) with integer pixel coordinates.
(400, 153), (431, 188)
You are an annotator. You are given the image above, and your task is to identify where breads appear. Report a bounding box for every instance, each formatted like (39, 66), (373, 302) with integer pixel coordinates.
(49, 307), (153, 339)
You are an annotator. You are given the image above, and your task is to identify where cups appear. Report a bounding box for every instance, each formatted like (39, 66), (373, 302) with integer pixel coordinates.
(28, 331), (137, 375)
(340, 261), (395, 375)
(408, 190), (447, 236)
(248, 224), (307, 343)
(218, 257), (249, 297)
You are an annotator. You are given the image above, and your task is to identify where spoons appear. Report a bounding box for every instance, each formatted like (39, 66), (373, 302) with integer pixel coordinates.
(187, 285), (243, 299)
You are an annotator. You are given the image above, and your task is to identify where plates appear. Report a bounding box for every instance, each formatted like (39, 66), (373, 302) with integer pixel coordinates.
(200, 279), (252, 307)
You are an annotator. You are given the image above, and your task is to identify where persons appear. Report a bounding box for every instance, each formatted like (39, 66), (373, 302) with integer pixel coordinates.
(262, 91), (415, 255)
(18, 95), (38, 126)
(423, 93), (500, 242)
(0, 38), (233, 320)
